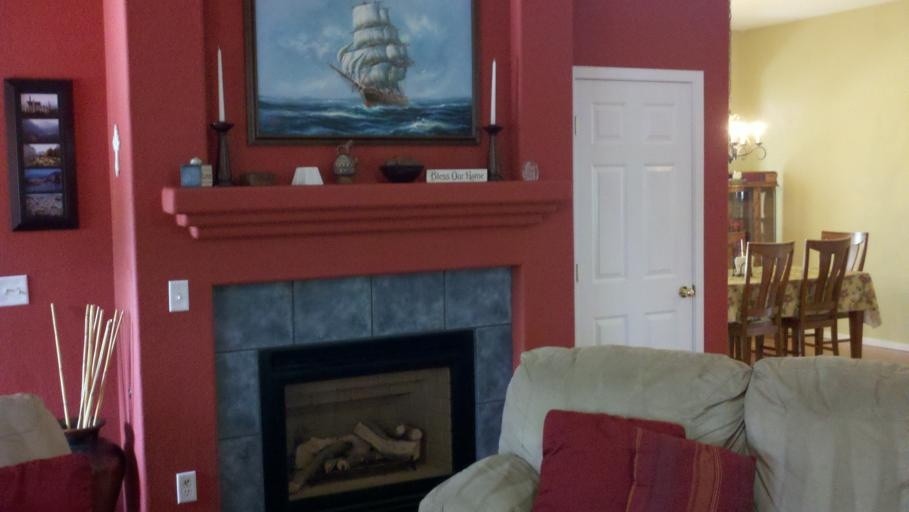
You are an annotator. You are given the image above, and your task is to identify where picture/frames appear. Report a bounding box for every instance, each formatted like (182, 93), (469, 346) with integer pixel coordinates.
(4, 77), (79, 231)
(241, 0), (482, 144)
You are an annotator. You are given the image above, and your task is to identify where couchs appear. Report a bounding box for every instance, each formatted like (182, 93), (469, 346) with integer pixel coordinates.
(417, 345), (909, 511)
(0, 393), (70, 468)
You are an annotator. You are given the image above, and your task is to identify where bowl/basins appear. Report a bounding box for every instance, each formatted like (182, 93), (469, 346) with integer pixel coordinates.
(378, 165), (428, 182)
(291, 166), (324, 185)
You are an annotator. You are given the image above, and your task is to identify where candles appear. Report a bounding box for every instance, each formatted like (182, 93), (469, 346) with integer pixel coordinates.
(490, 58), (497, 127)
(218, 46), (225, 123)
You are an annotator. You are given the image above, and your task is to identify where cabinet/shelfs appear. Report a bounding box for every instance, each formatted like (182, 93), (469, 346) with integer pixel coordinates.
(729, 171), (778, 269)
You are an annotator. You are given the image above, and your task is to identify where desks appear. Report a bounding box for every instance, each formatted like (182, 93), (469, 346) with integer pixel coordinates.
(728, 266), (882, 358)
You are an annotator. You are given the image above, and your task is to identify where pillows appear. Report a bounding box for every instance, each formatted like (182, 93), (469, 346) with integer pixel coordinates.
(533, 409), (686, 512)
(0, 456), (94, 511)
(623, 425), (758, 511)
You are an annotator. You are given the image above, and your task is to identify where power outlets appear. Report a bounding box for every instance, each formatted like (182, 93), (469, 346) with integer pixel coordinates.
(176, 469), (198, 502)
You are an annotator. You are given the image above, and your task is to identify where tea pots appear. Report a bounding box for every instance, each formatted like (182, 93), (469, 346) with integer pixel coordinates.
(333, 140), (360, 177)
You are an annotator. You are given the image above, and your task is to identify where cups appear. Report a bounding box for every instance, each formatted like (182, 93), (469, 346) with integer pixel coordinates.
(521, 160), (540, 182)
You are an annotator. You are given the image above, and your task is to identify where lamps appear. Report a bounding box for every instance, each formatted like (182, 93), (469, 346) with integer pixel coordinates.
(728, 113), (766, 164)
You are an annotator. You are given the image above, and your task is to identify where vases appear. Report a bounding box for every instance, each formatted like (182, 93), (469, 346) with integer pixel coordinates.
(56, 417), (126, 512)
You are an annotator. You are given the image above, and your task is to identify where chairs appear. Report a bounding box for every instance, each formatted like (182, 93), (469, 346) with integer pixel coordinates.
(782, 236), (852, 358)
(730, 242), (795, 365)
(820, 229), (868, 358)
